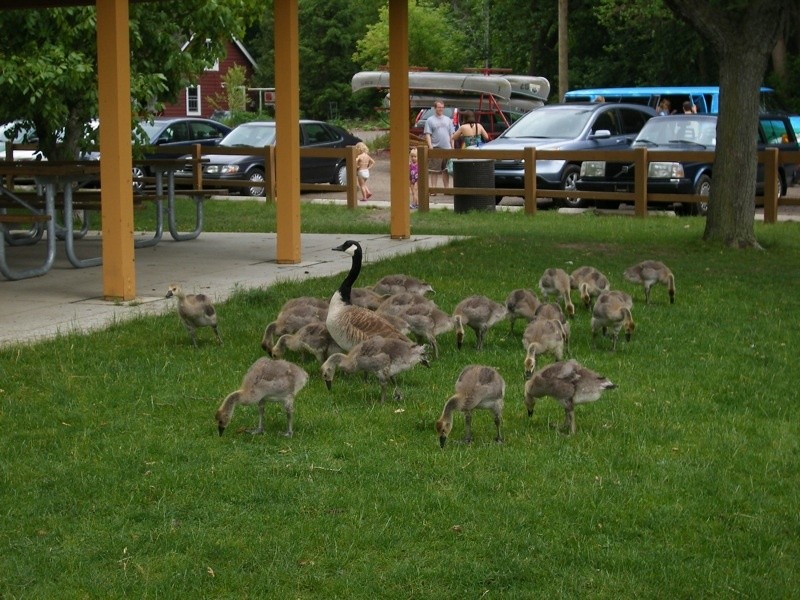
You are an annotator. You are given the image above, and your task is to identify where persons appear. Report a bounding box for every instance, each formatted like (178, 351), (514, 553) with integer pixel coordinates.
(424, 100), (455, 196)
(656, 99), (676, 116)
(452, 111), (488, 148)
(355, 142), (375, 201)
(595, 96), (605, 103)
(683, 101), (697, 115)
(409, 148), (418, 209)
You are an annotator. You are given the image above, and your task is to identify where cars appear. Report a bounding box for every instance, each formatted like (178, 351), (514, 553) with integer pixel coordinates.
(576, 110), (800, 217)
(174, 117), (363, 197)
(475, 100), (665, 208)
(413, 108), (518, 142)
(0, 117), (234, 193)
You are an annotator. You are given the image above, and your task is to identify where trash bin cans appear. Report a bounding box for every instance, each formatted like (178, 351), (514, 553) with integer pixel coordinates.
(453, 158), (495, 213)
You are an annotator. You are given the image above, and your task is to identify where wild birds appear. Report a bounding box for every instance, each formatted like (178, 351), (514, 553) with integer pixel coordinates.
(535, 303), (570, 344)
(326, 240), (431, 384)
(539, 268), (575, 319)
(452, 295), (508, 350)
(569, 266), (610, 309)
(506, 288), (541, 336)
(622, 260), (675, 305)
(271, 322), (344, 364)
(261, 297), (329, 357)
(524, 359), (618, 435)
(591, 290), (635, 350)
(165, 284), (223, 347)
(215, 357), (309, 439)
(436, 365), (506, 448)
(349, 274), (451, 361)
(522, 319), (564, 381)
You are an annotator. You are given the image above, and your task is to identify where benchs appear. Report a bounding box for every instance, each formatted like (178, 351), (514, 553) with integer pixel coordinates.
(0, 187), (231, 223)
(194, 130), (219, 140)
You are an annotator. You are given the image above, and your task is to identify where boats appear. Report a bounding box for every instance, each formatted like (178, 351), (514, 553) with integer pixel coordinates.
(449, 74), (551, 103)
(350, 70), (513, 101)
(382, 92), (546, 117)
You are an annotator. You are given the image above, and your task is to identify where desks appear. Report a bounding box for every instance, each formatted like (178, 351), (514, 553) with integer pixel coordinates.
(0, 167), (102, 280)
(10, 159), (211, 249)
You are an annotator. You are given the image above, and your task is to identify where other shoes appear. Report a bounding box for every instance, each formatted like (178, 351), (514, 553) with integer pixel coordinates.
(366, 193), (372, 199)
(409, 204), (416, 209)
(360, 199), (368, 201)
(430, 193), (437, 196)
(445, 193), (452, 195)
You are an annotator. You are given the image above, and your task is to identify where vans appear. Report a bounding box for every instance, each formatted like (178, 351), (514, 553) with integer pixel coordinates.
(563, 85), (800, 145)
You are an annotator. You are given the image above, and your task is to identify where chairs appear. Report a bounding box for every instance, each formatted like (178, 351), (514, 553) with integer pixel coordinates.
(309, 131), (328, 143)
(168, 129), (184, 142)
(261, 131), (272, 144)
(677, 128), (695, 141)
